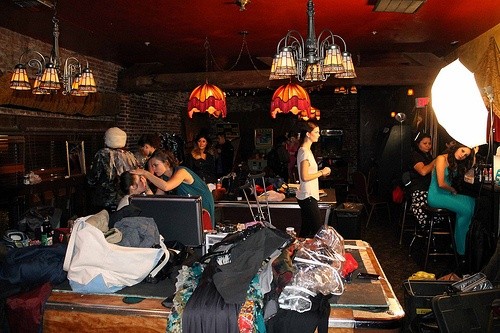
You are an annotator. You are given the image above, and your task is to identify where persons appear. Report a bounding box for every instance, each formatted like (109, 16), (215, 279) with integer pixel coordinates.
(410, 134), (443, 238)
(185, 133), (235, 183)
(271, 134), (300, 182)
(427, 143), (475, 260)
(296, 123), (330, 238)
(80, 127), (215, 230)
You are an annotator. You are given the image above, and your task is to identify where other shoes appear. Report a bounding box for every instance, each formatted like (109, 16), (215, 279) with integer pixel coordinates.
(459, 256), (469, 269)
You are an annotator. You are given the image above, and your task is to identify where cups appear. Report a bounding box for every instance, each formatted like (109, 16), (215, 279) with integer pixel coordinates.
(286, 227), (295, 236)
(237, 197), (243, 201)
(225, 178), (231, 186)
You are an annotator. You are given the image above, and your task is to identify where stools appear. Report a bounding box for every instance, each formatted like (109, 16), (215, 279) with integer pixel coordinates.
(398, 188), (459, 272)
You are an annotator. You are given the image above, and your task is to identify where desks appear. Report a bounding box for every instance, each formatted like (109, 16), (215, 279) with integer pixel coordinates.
(45, 237), (404, 333)
(211, 185), (337, 238)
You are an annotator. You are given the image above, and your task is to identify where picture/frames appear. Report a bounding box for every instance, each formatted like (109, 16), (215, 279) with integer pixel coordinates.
(65, 141), (87, 179)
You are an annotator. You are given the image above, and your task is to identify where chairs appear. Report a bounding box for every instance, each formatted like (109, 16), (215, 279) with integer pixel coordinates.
(356, 169), (393, 229)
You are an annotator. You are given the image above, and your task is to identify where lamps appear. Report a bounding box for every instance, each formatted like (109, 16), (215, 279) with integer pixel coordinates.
(271, 81), (313, 121)
(298, 105), (322, 121)
(334, 80), (359, 94)
(268, 0), (357, 80)
(187, 35), (227, 120)
(9, 0), (97, 97)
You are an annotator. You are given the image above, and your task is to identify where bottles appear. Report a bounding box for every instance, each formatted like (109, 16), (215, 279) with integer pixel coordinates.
(24, 176), (31, 185)
(496, 169), (500, 186)
(40, 216), (54, 246)
(217, 179), (222, 189)
(478, 161), (492, 182)
(202, 177), (206, 183)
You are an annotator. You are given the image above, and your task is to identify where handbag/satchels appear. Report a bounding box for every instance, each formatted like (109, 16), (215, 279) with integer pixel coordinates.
(64, 215), (170, 294)
(148, 240), (184, 284)
